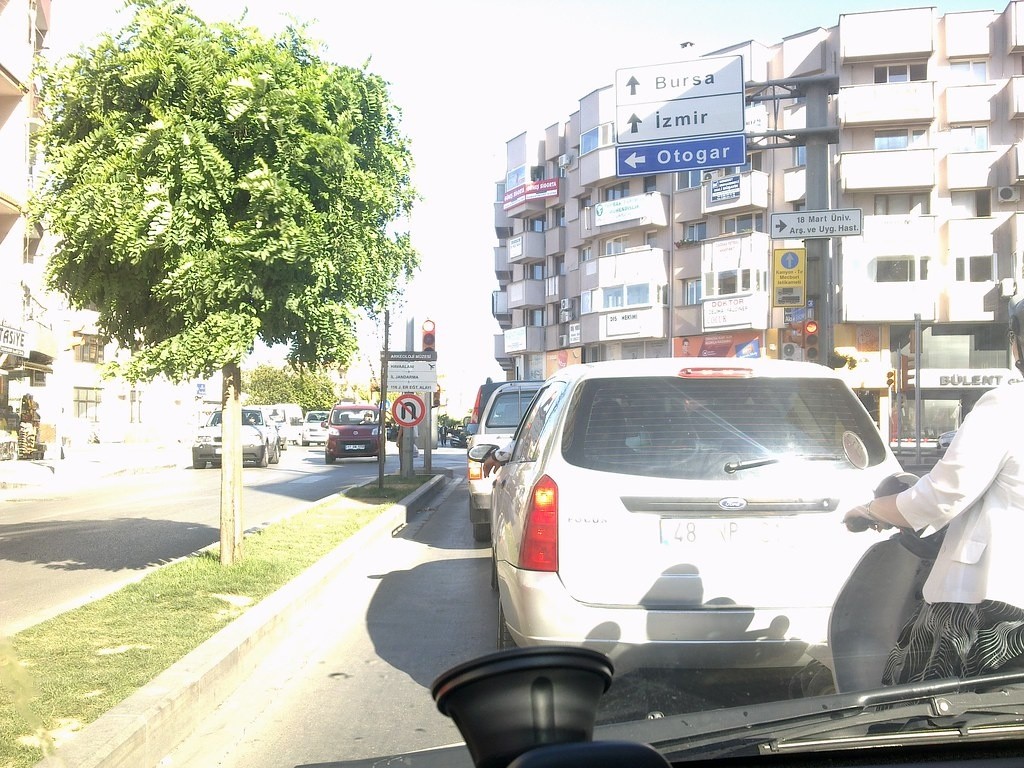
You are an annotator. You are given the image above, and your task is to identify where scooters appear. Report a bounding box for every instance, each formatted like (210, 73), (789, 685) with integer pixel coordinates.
(827, 473), (950, 692)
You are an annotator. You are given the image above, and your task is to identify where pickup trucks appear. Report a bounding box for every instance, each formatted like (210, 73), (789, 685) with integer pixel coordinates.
(467, 383), (548, 542)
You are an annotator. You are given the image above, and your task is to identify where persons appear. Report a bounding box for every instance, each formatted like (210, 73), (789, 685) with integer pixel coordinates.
(247, 414), (260, 425)
(360, 414), (372, 424)
(678, 339), (694, 356)
(483, 442), (516, 478)
(843, 296), (1024, 725)
(4, 406), (20, 420)
(439, 424), (448, 446)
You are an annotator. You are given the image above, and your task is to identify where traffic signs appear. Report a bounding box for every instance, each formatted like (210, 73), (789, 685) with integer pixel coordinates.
(770, 207), (863, 239)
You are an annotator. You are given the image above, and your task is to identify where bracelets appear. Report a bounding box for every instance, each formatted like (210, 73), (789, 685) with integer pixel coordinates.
(492, 454), (499, 461)
(866, 500), (874, 520)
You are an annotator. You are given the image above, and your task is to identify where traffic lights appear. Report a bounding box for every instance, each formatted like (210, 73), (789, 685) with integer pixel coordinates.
(422, 320), (435, 352)
(908, 356), (915, 389)
(805, 320), (819, 360)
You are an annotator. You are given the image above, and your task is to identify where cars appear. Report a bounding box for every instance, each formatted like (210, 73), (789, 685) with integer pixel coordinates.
(301, 411), (331, 446)
(325, 402), (391, 464)
(464, 378), (547, 434)
(451, 434), (467, 448)
(938, 429), (958, 448)
(387, 426), (400, 442)
(246, 403), (304, 450)
(468, 356), (937, 674)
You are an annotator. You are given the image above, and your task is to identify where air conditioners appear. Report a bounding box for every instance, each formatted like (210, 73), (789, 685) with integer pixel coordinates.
(999, 278), (1016, 297)
(561, 311), (572, 323)
(561, 298), (573, 310)
(559, 335), (569, 346)
(559, 154), (570, 165)
(704, 170), (720, 181)
(782, 343), (804, 362)
(998, 185), (1022, 202)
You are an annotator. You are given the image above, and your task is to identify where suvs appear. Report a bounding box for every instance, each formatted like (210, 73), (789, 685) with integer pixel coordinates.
(192, 407), (280, 468)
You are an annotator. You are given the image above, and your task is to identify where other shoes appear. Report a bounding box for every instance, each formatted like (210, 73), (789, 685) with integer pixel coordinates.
(444, 442), (446, 445)
(441, 444), (443, 447)
